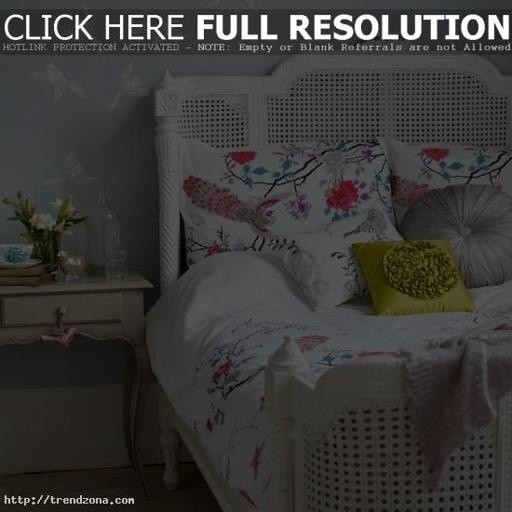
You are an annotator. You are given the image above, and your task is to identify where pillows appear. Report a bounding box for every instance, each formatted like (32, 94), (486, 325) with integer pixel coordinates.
(172, 135), (512, 316)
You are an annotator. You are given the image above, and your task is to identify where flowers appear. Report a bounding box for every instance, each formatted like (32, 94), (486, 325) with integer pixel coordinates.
(3, 185), (91, 281)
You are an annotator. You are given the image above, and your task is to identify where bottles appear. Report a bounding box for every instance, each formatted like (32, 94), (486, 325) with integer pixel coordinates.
(105, 231), (129, 282)
(85, 190), (119, 277)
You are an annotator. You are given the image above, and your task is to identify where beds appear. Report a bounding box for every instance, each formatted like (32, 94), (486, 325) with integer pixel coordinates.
(130, 52), (512, 511)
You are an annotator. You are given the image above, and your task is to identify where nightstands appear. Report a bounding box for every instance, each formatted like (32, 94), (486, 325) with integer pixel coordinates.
(1, 270), (155, 504)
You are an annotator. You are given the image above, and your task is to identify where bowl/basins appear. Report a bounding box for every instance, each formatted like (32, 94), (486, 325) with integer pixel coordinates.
(0, 243), (34, 264)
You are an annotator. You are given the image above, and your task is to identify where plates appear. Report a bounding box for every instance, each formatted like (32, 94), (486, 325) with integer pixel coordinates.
(0, 259), (42, 270)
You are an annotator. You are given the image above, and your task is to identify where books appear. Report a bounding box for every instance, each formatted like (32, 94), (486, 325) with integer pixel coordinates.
(1, 263), (53, 277)
(1, 274), (55, 287)
